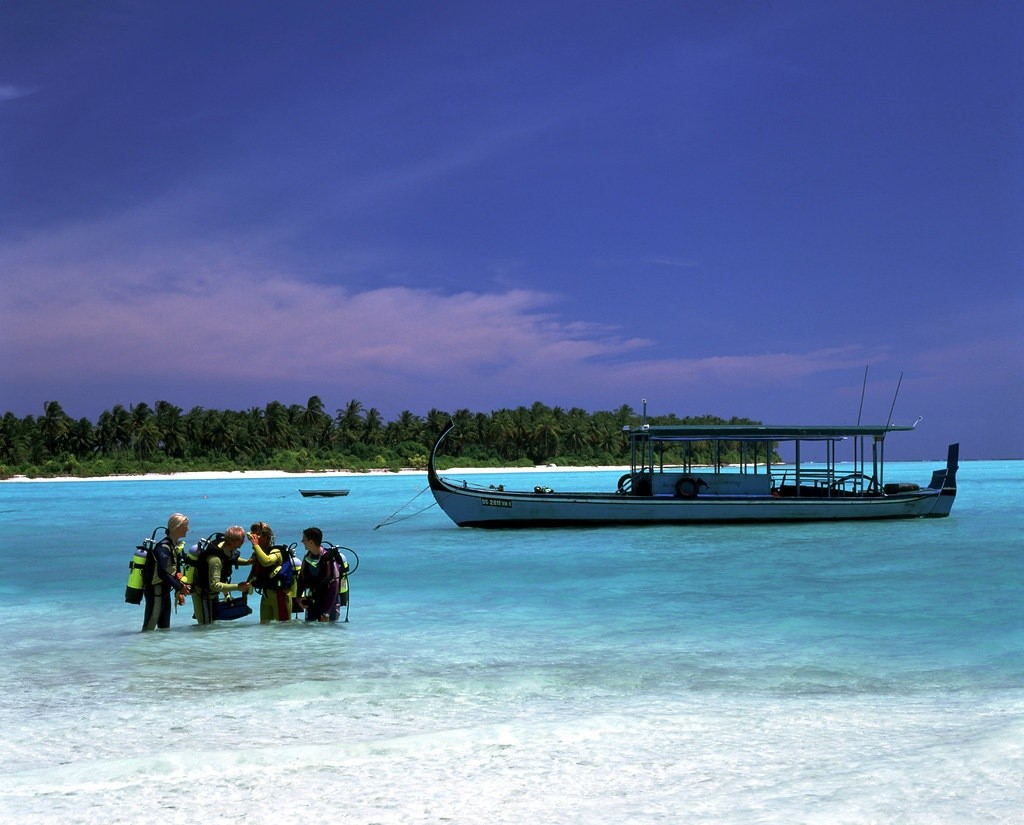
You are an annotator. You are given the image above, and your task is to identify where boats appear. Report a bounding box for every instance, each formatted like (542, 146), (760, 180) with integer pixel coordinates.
(427, 365), (960, 531)
(299, 489), (350, 498)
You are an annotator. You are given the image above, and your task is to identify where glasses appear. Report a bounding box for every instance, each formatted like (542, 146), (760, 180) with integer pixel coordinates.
(246, 532), (252, 539)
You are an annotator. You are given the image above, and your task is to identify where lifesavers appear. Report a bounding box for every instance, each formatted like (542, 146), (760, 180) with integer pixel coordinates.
(617, 474), (632, 495)
(676, 477), (698, 499)
(884, 483), (920, 492)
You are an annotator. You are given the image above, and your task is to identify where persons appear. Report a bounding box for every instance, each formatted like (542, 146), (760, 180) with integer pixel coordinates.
(246, 521), (294, 622)
(296, 527), (341, 622)
(142, 513), (191, 633)
(192, 525), (253, 626)
